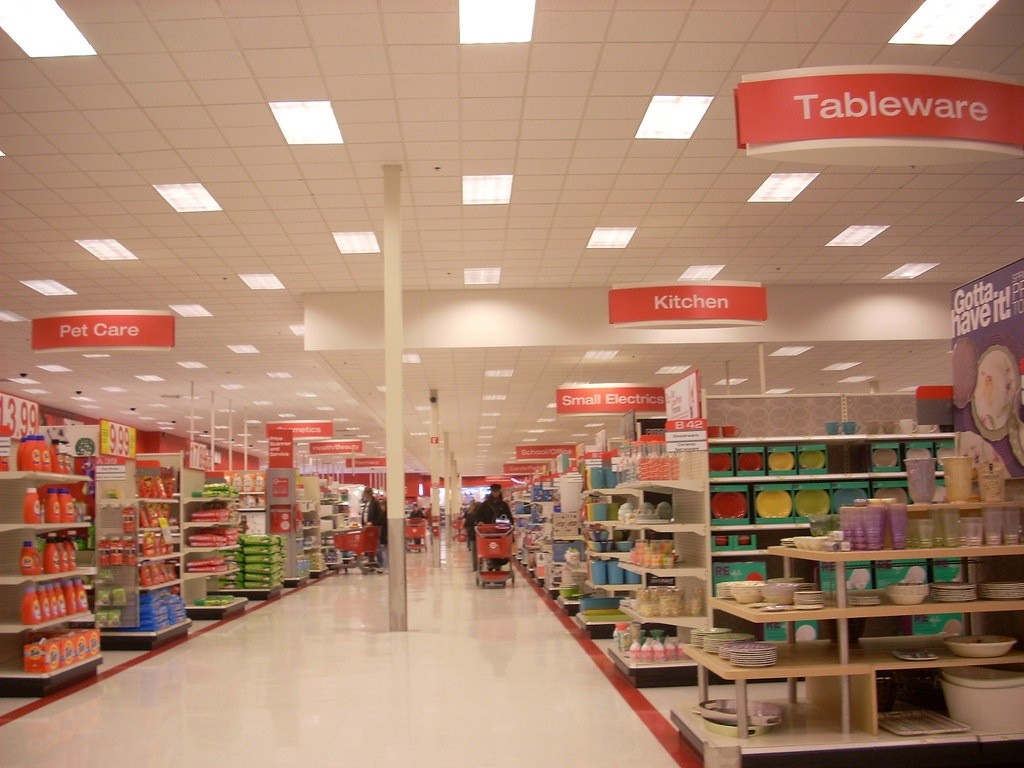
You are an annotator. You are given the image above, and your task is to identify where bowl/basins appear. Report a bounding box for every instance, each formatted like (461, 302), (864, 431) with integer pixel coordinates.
(715, 577), (801, 610)
(583, 529), (633, 552)
(942, 635), (1017, 657)
(793, 536), (830, 551)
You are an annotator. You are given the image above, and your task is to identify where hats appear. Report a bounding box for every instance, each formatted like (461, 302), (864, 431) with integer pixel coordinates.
(490, 484), (501, 491)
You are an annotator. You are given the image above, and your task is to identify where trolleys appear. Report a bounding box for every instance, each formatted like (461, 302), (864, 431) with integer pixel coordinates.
(473, 521), (515, 589)
(404, 517), (426, 552)
(333, 520), (382, 575)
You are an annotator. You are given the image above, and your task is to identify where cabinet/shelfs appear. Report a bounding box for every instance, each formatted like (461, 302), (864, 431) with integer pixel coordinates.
(605, 432), (960, 690)
(0, 421), (445, 698)
(671, 456), (1024, 767)
(491, 408), (677, 640)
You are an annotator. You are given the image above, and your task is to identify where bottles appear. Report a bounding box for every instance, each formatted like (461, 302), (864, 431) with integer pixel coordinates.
(617, 440), (691, 480)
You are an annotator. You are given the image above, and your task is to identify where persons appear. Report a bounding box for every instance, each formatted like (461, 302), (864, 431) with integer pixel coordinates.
(463, 499), (481, 571)
(362, 488), (388, 575)
(477, 484), (514, 585)
(409, 503), (432, 552)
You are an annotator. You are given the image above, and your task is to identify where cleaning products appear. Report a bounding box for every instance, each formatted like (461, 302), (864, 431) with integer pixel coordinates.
(100, 476), (176, 587)
(15, 435), (102, 673)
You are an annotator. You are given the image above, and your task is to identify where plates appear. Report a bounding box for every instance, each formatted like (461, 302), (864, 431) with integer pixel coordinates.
(847, 581), (1024, 606)
(712, 487), (909, 520)
(690, 628), (778, 667)
(874, 447), (956, 468)
(709, 450), (826, 470)
(780, 538), (796, 548)
(690, 698), (785, 737)
(792, 591), (825, 610)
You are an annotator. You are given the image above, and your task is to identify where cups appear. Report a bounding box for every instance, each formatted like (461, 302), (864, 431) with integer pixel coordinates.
(825, 421), (860, 435)
(903, 457), (1006, 503)
(905, 519), (934, 548)
(983, 505), (1020, 545)
(899, 419), (937, 433)
(959, 517), (983, 546)
(809, 514), (840, 537)
(864, 421), (900, 434)
(928, 507), (960, 547)
(707, 426), (741, 438)
(630, 540), (676, 568)
(840, 507), (908, 550)
